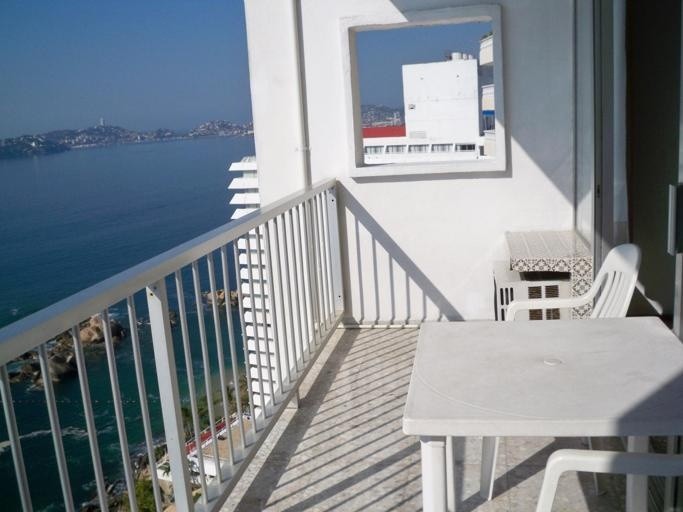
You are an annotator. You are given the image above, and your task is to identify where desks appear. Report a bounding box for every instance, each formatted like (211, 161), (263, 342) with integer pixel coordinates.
(402, 312), (681, 510)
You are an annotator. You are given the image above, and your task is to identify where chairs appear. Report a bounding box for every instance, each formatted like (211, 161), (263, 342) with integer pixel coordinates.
(475, 240), (642, 502)
